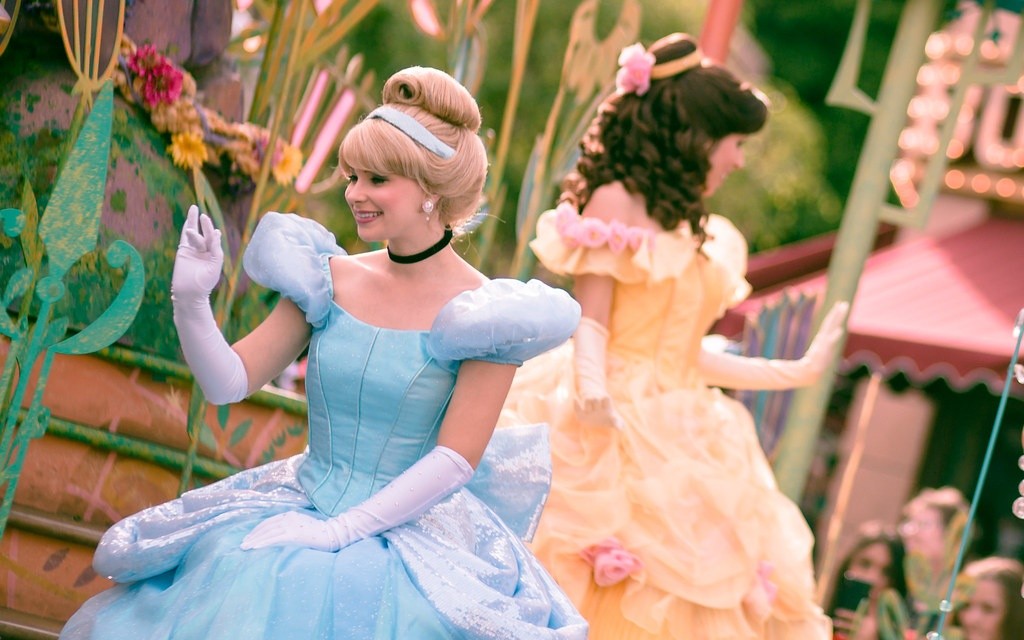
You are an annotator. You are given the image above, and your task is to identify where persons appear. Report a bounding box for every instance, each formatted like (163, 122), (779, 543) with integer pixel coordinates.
(494, 29), (850, 640)
(53, 64), (589, 640)
(821, 487), (1024, 640)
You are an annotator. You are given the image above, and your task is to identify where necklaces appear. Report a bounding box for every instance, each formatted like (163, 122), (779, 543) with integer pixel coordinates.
(386, 223), (453, 264)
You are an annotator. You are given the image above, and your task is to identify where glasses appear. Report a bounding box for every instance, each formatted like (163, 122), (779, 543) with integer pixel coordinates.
(856, 556), (892, 578)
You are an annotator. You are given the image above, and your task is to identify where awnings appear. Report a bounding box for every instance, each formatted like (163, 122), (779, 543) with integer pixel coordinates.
(707, 201), (1024, 405)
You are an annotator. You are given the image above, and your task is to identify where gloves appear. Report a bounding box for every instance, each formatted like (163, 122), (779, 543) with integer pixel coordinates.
(240, 444), (474, 551)
(170, 204), (250, 405)
(571, 318), (622, 430)
(701, 301), (851, 390)
